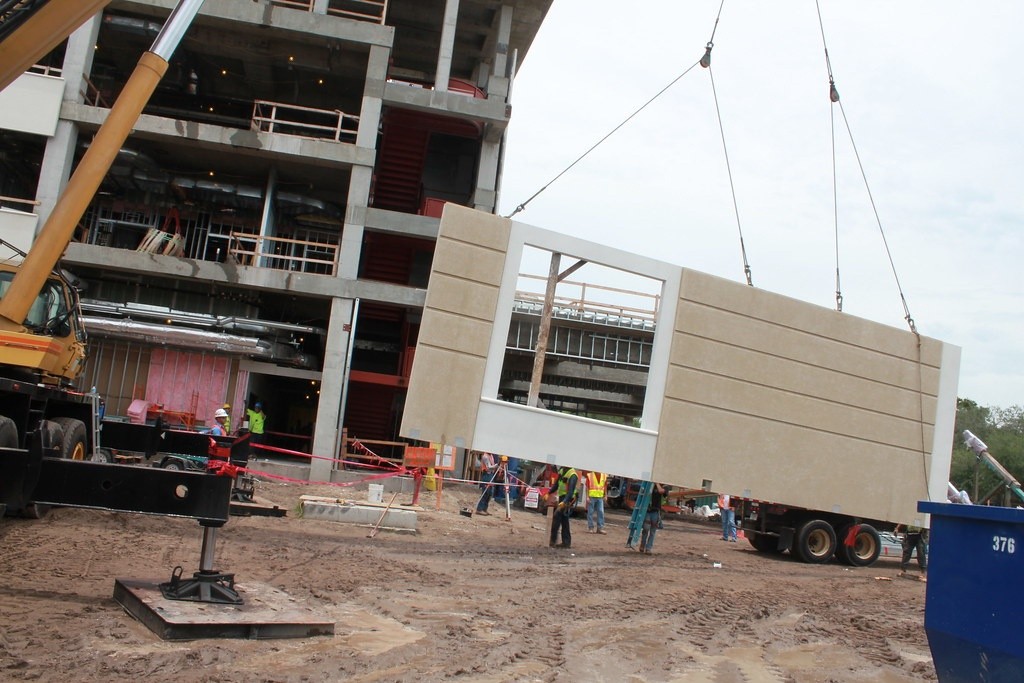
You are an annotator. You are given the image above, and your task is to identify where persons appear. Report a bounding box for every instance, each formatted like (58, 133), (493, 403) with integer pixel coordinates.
(210, 408), (227, 436)
(246, 402), (268, 461)
(893, 523), (928, 574)
(639, 482), (668, 554)
(476, 452), (498, 516)
(585, 471), (608, 534)
(690, 498), (696, 513)
(543, 466), (579, 547)
(717, 493), (737, 542)
(223, 404), (230, 432)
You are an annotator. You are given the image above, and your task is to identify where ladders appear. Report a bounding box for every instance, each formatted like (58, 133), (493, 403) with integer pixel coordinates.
(626, 481), (654, 547)
(187, 388), (199, 431)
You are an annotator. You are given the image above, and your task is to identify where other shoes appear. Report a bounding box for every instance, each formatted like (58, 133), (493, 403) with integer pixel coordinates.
(550, 540), (556, 546)
(897, 569), (906, 577)
(476, 510), (487, 515)
(486, 511), (492, 516)
(640, 543), (645, 552)
(919, 575), (927, 582)
(597, 530), (607, 535)
(556, 543), (571, 548)
(720, 537), (726, 541)
(729, 539), (735, 542)
(589, 528), (593, 534)
(645, 549), (652, 555)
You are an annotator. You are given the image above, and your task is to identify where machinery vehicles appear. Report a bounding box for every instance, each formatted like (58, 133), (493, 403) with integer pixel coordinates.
(737, 496), (883, 568)
(1, 0), (340, 650)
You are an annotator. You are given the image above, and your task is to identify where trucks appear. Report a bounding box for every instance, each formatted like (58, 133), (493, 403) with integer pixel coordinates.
(914, 496), (1024, 683)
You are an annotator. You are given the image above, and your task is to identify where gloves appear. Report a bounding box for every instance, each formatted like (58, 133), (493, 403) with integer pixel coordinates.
(543, 493), (549, 501)
(893, 528), (898, 536)
(558, 502), (565, 509)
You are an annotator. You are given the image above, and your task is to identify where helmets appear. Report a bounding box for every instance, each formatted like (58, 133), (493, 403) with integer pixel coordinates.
(255, 402), (261, 408)
(215, 409), (228, 417)
(223, 404), (230, 408)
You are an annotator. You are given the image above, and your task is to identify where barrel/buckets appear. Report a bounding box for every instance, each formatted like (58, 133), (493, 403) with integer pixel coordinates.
(367, 483), (384, 505)
